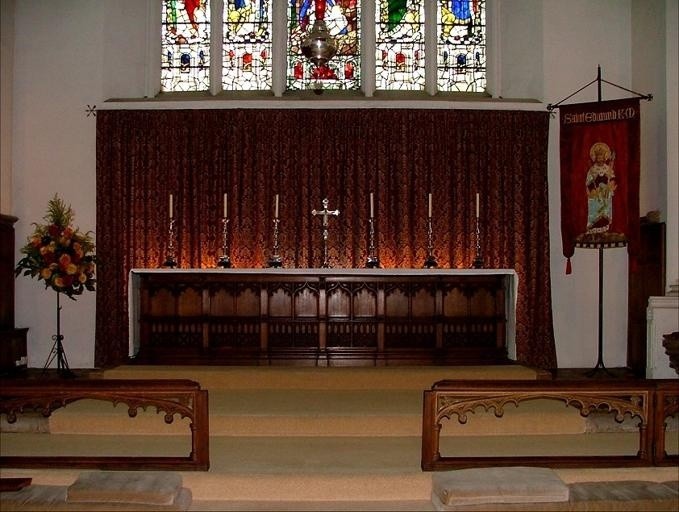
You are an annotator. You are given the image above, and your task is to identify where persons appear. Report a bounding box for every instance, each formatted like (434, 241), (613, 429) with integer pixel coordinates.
(585, 147), (616, 235)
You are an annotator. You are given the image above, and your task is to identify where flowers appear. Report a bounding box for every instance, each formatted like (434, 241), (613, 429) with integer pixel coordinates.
(10, 192), (108, 302)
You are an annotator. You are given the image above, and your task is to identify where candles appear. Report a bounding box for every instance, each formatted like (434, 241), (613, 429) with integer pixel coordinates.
(369, 192), (375, 219)
(222, 192), (228, 219)
(273, 193), (281, 219)
(168, 193), (174, 218)
(427, 192), (434, 219)
(475, 193), (480, 219)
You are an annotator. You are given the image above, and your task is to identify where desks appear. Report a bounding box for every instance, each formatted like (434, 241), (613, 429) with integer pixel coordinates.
(124, 265), (520, 368)
